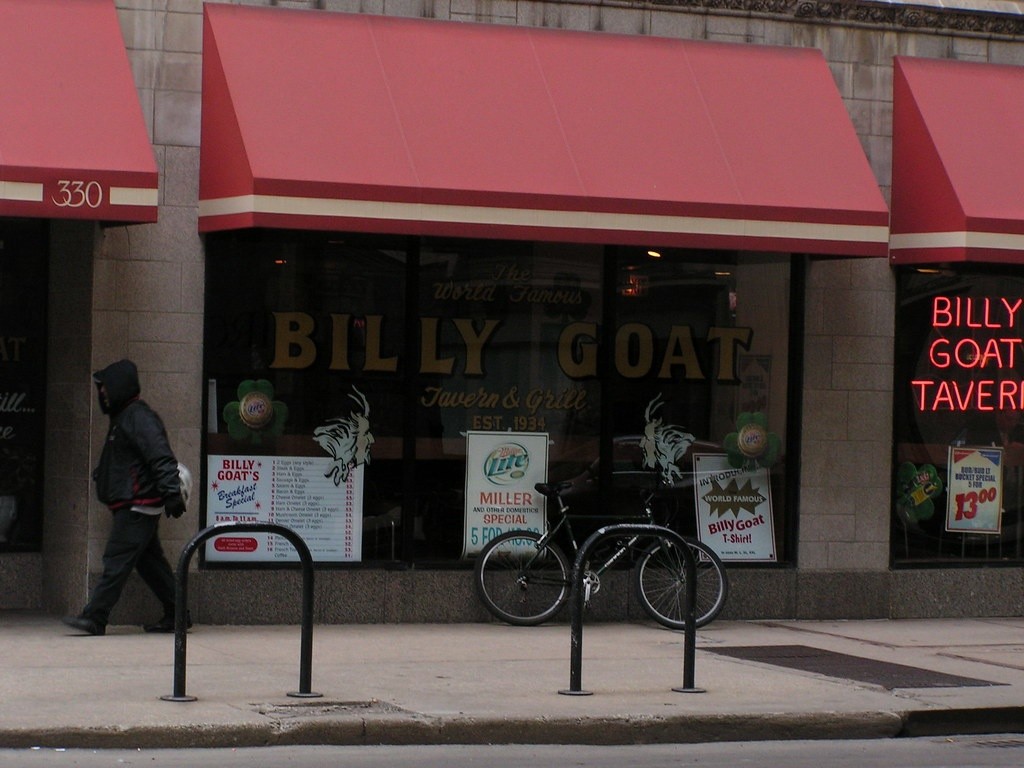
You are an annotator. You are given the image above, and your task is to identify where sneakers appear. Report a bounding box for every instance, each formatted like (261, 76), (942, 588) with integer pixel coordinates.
(144, 609), (193, 632)
(62, 614), (105, 635)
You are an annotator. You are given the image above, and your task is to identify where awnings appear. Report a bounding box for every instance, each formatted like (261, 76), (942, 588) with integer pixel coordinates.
(0, 0), (1024, 262)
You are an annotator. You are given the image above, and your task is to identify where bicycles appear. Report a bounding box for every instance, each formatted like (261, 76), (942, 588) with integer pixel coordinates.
(477, 460), (729, 630)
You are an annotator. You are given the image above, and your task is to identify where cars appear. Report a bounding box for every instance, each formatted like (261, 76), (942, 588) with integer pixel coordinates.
(549, 432), (790, 562)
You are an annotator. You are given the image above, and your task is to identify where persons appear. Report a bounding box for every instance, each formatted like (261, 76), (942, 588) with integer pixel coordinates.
(63, 360), (193, 634)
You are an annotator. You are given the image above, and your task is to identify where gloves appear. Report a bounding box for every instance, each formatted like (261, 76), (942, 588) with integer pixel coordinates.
(165, 494), (186, 518)
